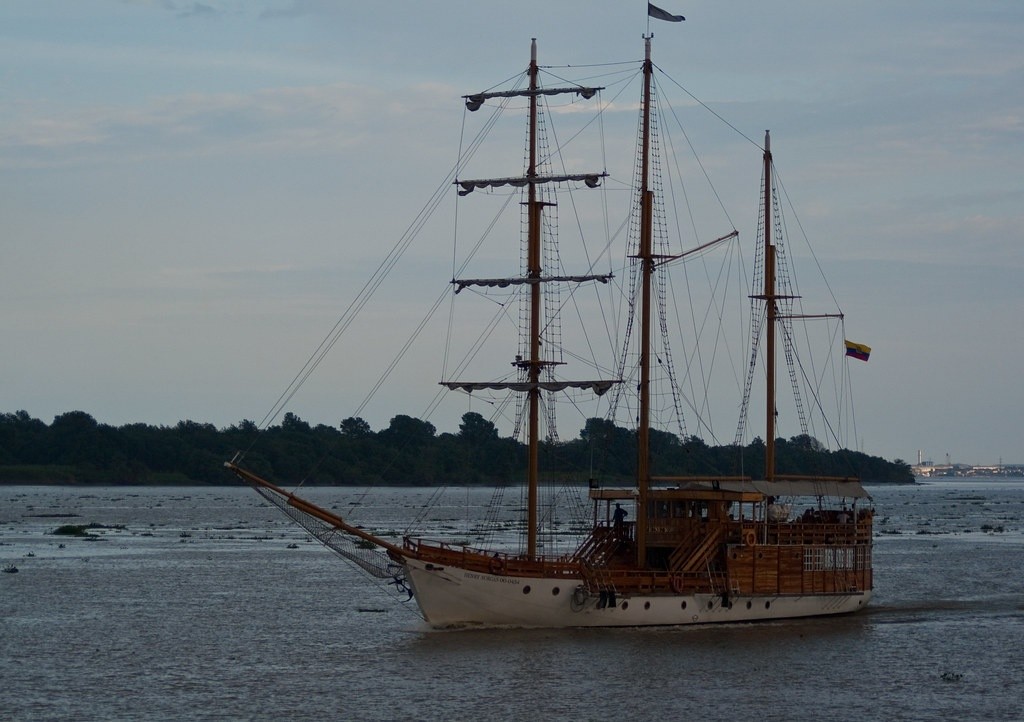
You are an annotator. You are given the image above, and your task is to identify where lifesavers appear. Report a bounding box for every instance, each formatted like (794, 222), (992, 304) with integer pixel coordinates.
(488, 556), (506, 575)
(745, 531), (757, 548)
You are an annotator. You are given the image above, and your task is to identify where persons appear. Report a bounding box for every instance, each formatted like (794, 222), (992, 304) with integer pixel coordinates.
(612, 502), (628, 537)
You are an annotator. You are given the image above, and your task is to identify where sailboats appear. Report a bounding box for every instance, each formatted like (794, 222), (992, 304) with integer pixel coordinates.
(218, 1), (877, 632)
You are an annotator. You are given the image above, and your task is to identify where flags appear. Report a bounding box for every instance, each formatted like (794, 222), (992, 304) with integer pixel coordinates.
(845, 340), (871, 361)
(648, 3), (685, 22)
